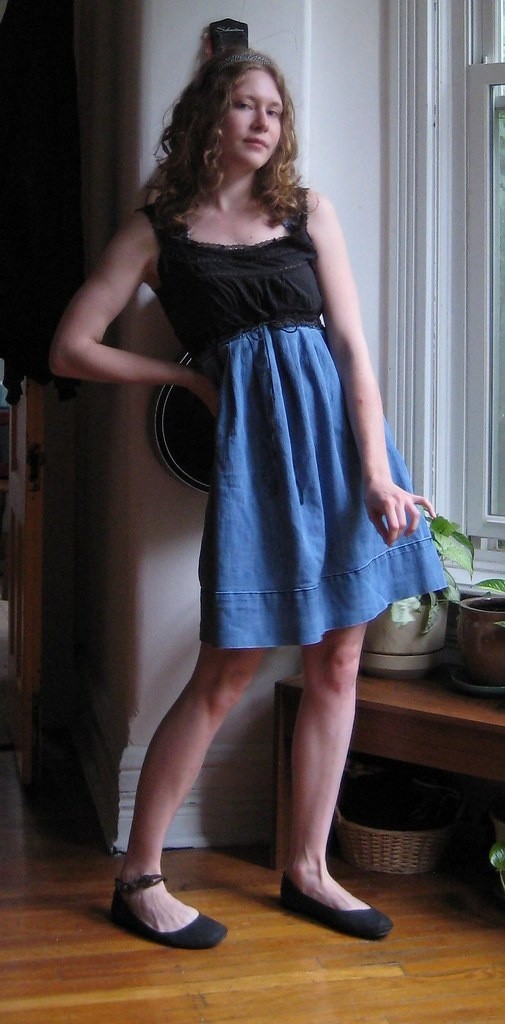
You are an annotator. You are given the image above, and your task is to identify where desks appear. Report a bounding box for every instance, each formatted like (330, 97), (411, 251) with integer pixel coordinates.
(273, 671), (504, 869)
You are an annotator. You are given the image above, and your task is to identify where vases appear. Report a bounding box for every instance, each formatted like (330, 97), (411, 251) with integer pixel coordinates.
(450, 595), (505, 697)
(488, 803), (505, 843)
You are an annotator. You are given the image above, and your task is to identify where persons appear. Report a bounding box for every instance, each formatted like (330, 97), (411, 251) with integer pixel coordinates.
(48, 47), (448, 948)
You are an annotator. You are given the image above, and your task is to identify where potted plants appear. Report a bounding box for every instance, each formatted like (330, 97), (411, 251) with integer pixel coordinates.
(358, 507), (475, 682)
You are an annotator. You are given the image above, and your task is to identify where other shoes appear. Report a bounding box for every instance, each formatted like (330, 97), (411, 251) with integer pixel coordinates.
(280, 869), (393, 938)
(111, 875), (228, 950)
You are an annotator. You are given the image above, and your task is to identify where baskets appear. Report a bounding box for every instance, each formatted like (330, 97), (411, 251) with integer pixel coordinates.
(334, 793), (458, 875)
